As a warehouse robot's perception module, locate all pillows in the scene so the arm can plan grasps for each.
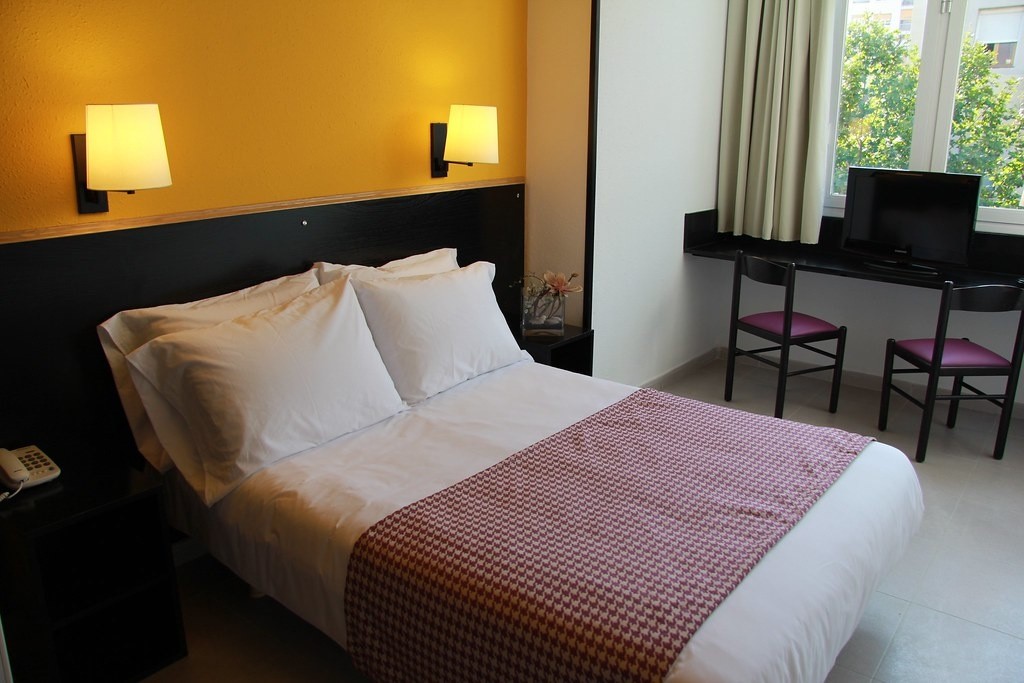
[98,267,320,477]
[123,273,409,507]
[351,263,535,404]
[313,248,460,284]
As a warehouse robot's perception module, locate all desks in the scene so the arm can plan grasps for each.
[687,207,1024,312]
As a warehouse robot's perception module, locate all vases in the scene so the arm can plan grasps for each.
[522,293,566,337]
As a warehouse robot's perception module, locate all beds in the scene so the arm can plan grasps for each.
[1,182,928,683]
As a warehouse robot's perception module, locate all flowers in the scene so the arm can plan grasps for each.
[522,268,584,327]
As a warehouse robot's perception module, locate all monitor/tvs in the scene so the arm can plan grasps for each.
[840,166,983,277]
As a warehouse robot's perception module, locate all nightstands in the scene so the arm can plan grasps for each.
[0,434,188,683]
[518,324,595,375]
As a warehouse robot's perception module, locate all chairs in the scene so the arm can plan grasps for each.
[723,250,847,422]
[878,279,1024,462]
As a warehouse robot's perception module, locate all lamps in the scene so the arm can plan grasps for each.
[430,104,501,178]
[68,102,172,213]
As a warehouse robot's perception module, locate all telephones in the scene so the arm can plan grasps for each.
[0,445,61,491]
